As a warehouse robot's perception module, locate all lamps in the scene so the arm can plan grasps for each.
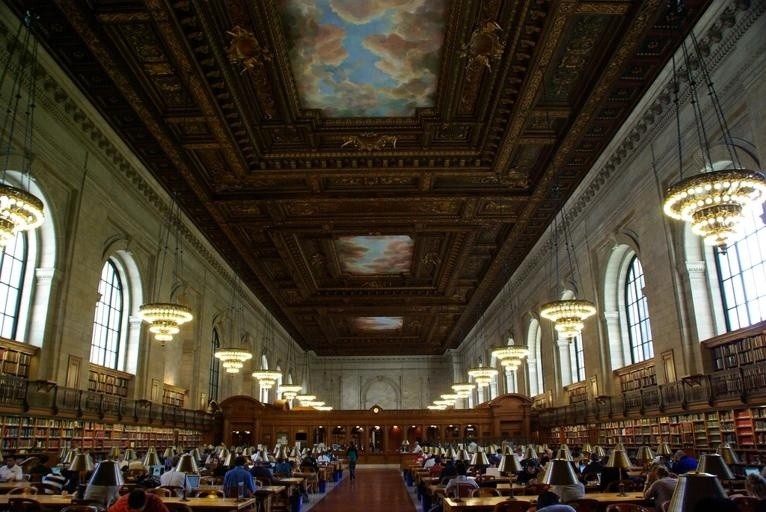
[139,192,193,347]
[0,11,46,245]
[0,442,348,512]
[540,171,597,344]
[215,348,332,416]
[400,441,766,512]
[663,29,765,255]
[427,344,530,410]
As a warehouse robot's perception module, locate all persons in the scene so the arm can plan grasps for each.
[1,439,360,511]
[402,439,766,511]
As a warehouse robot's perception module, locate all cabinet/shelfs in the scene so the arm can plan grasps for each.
[530,322,766,451]
[0,338,215,447]
